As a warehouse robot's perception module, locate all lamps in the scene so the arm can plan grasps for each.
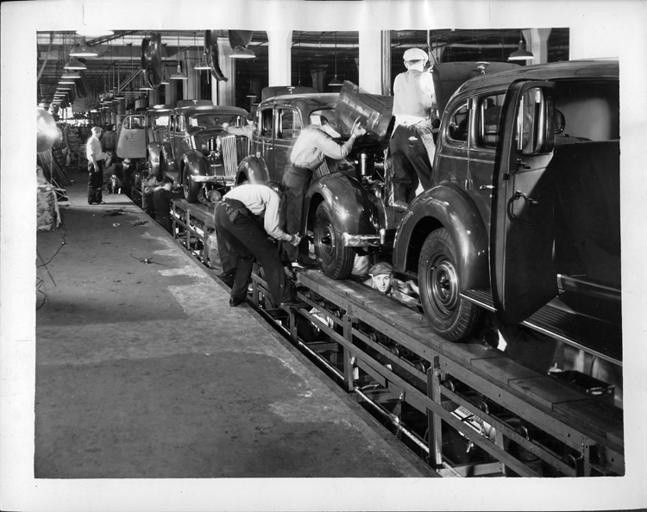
[51,14,256,97]
[328,32,344,88]
[507,30,535,62]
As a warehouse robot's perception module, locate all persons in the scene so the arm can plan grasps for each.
[386,47,440,210]
[281,109,368,269]
[85,122,132,206]
[213,181,308,308]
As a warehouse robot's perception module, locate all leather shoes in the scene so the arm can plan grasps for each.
[297,254,321,269]
[279,301,308,308]
[229,289,248,307]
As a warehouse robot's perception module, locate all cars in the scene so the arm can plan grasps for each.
[388,59,620,368]
[116,54,394,283]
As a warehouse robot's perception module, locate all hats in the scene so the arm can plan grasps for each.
[402,47,429,63]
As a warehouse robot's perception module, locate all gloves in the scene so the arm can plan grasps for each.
[290,232,301,247]
